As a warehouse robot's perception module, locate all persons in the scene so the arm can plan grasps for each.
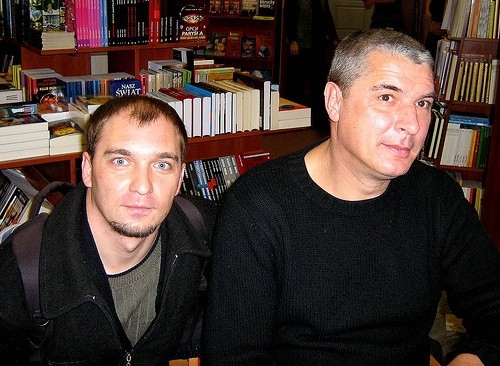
[198,26,500,366]
[0,95,216,366]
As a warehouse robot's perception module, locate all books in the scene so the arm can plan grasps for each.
[0,0,312,236]
[417,1,500,220]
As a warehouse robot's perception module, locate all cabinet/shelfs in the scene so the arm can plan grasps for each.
[0,0,500,250]
